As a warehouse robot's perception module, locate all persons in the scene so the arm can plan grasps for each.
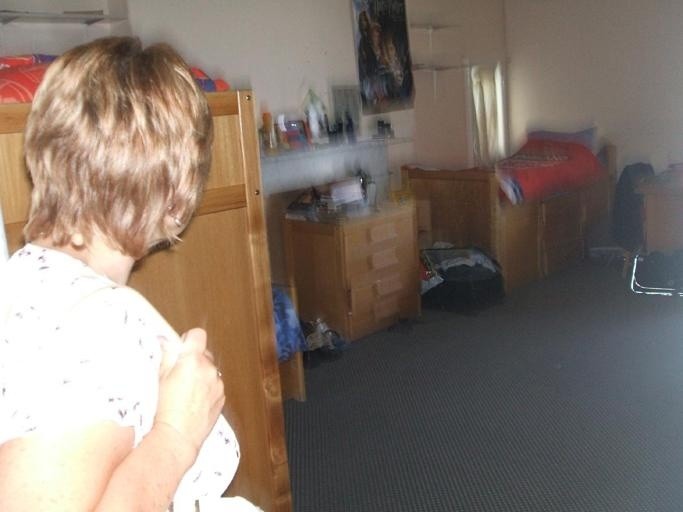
[0,34,270,512]
[356,9,373,84]
[364,22,389,84]
[380,35,406,109]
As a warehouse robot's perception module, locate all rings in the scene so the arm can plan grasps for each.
[216,366,222,376]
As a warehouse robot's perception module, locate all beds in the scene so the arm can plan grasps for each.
[401,126,615,295]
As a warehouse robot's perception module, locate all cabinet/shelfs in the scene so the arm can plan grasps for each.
[4,91,292,511]
[286,200,423,344]
[261,122,414,214]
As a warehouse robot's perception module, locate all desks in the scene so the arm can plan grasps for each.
[632,168,683,256]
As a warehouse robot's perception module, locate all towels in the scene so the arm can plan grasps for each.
[527,127,610,157]
